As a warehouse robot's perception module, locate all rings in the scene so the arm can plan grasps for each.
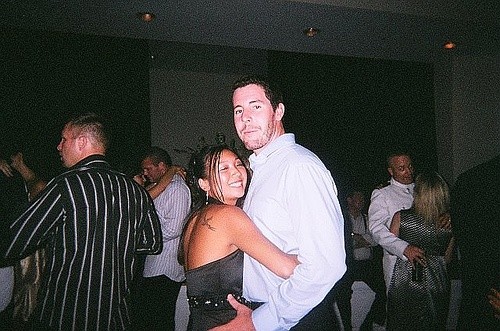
[449,219,451,222]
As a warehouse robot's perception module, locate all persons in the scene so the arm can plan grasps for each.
[0,136,62,331]
[387,169,457,331]
[336,185,388,331]
[367,148,454,289]
[0,131,24,331]
[124,159,187,200]
[126,146,191,331]
[208,75,347,331]
[0,112,163,331]
[177,142,300,331]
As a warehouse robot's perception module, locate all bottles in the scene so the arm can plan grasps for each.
[413,249,423,282]
